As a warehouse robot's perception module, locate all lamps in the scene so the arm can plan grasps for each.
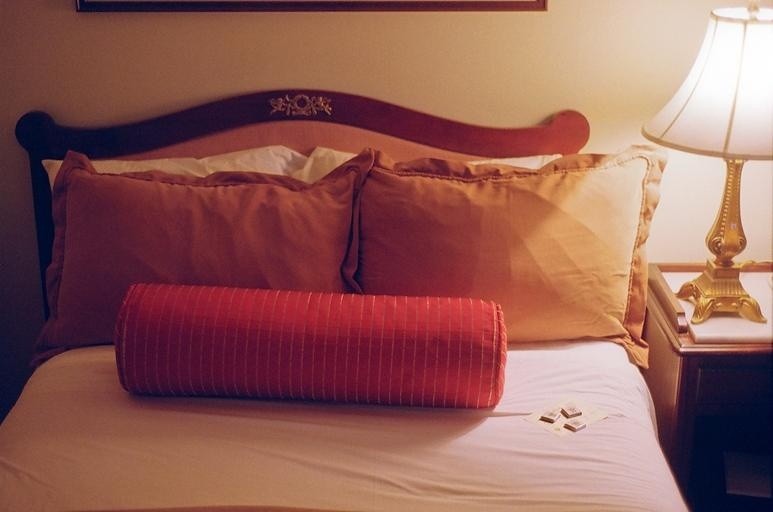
[641,7,773,324]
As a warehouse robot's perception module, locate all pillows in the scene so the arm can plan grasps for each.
[30,147,374,361]
[360,144,668,368]
[114,283,507,410]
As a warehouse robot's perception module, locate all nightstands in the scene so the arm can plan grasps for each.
[640,263,773,511]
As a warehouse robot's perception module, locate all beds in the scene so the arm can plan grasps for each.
[0,89,688,511]
